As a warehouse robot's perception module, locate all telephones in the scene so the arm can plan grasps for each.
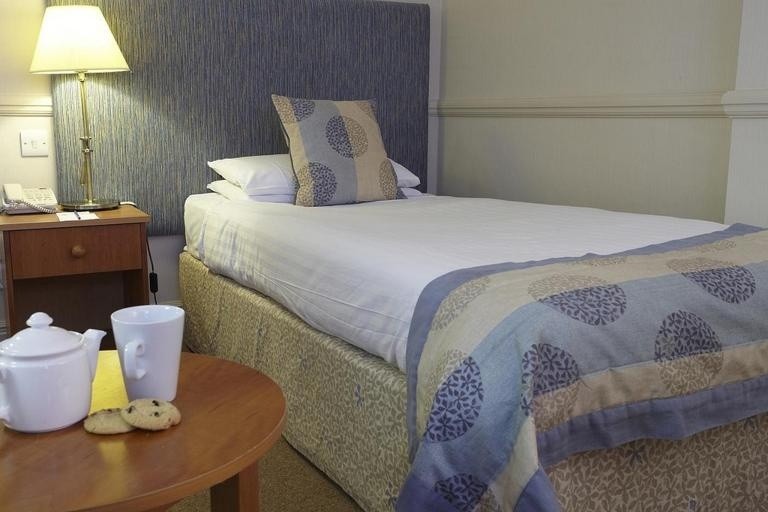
[1,182,59,215]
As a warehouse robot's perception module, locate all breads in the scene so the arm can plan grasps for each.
[122,398,181,431]
[84,408,136,435]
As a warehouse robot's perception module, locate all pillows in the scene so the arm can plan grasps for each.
[206,180,423,202]
[207,154,421,196]
[271,94,409,206]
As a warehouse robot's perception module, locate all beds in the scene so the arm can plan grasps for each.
[177,192,767,511]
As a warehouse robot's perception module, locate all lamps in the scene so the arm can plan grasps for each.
[29,4,130,211]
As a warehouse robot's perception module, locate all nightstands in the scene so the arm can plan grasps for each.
[0,205,150,338]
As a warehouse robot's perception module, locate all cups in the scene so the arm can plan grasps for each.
[110,304,185,403]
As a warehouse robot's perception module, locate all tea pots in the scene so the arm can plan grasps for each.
[0,311,107,434]
